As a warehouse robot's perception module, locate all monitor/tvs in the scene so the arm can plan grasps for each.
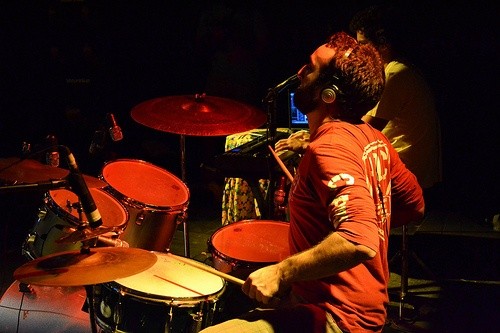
[287,87,310,129]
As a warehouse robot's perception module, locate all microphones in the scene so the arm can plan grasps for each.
[64,146,103,229]
[55,223,130,248]
[108,113,124,141]
[45,135,60,167]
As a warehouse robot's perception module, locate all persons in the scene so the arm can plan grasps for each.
[241,32,426,333]
[222,129,273,227]
[275,20,441,185]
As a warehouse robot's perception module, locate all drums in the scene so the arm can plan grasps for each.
[209,219,291,281]
[99,158,191,251]
[93,251,230,333]
[21,190,130,263]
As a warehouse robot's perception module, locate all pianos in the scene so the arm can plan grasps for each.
[216,132,306,221]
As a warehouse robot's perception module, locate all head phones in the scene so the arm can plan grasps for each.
[319,43,359,107]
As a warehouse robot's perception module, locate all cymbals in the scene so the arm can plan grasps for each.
[13,246,158,286]
[0,155,109,190]
[131,95,269,138]
